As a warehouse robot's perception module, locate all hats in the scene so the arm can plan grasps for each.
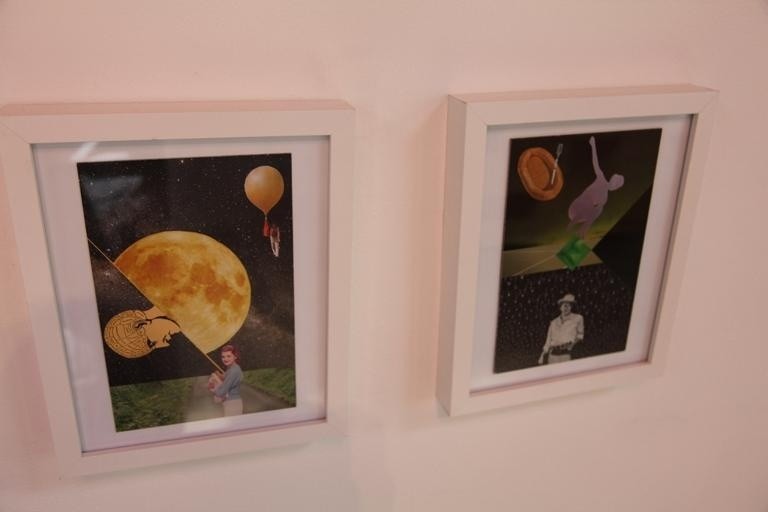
[556,294,579,306]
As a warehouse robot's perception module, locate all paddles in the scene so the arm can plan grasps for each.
[551,142,563,184]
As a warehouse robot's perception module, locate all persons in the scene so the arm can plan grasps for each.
[269,219,283,258]
[567,135,625,240]
[101,300,184,362]
[208,342,246,419]
[536,292,586,368]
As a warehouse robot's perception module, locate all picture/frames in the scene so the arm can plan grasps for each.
[0,99,357,479]
[436,85,720,418]
